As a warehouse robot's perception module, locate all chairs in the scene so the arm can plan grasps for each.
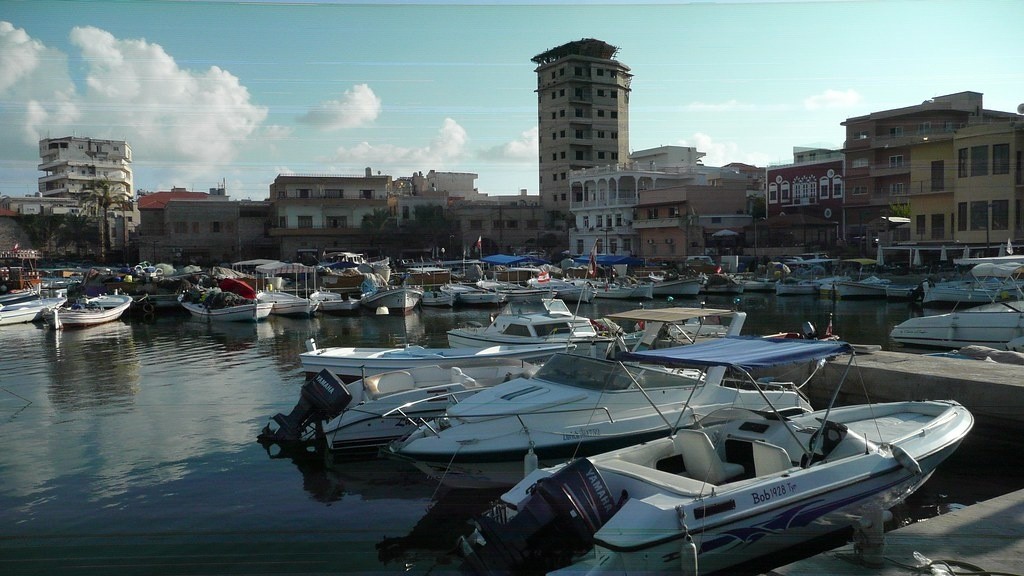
[753,439,794,478]
[677,429,744,484]
[450,367,483,389]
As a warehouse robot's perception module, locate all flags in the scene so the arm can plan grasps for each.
[476,236,482,248]
[11,243,18,252]
[538,271,550,282]
[589,244,600,275]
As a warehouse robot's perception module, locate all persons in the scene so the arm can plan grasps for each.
[101,266,148,285]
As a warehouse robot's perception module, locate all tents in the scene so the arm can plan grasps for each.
[194,253,644,298]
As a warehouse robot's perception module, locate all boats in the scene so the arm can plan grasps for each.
[400,305,815,490]
[297,336,577,389]
[603,304,736,350]
[446,294,599,361]
[254,357,548,457]
[888,262,1024,349]
[460,332,975,576]
[0,253,1024,328]
